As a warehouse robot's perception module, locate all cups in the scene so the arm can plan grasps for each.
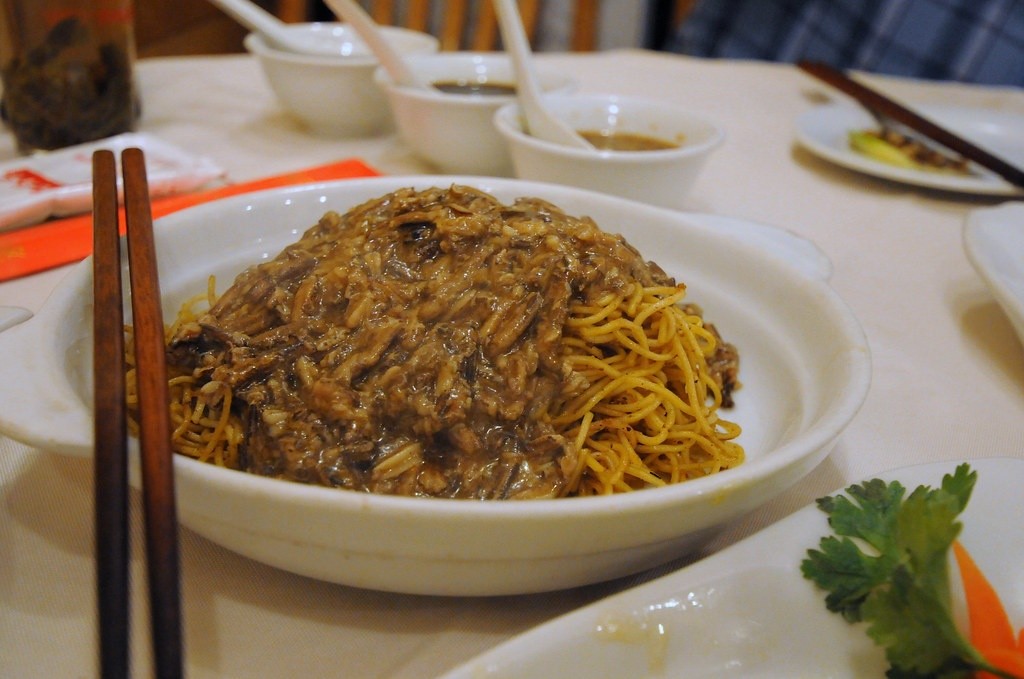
[0,0,141,154]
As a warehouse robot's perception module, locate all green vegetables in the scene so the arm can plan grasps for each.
[800,462,1024,678]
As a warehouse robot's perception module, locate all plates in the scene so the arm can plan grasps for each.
[960,201,1024,344]
[0,131,227,228]
[424,458,1024,678]
[792,102,1024,199]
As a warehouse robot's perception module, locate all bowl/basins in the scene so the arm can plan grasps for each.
[0,177,871,597]
[370,48,580,177]
[242,21,438,138]
[492,91,723,212]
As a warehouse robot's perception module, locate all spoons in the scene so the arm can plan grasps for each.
[491,0,595,151]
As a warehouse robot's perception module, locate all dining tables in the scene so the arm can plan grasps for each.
[1,53,1022,679]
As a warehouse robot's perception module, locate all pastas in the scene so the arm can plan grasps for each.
[123,269,747,496]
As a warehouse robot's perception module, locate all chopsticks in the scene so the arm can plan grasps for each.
[793,54,1024,190]
[89,147,185,679]
